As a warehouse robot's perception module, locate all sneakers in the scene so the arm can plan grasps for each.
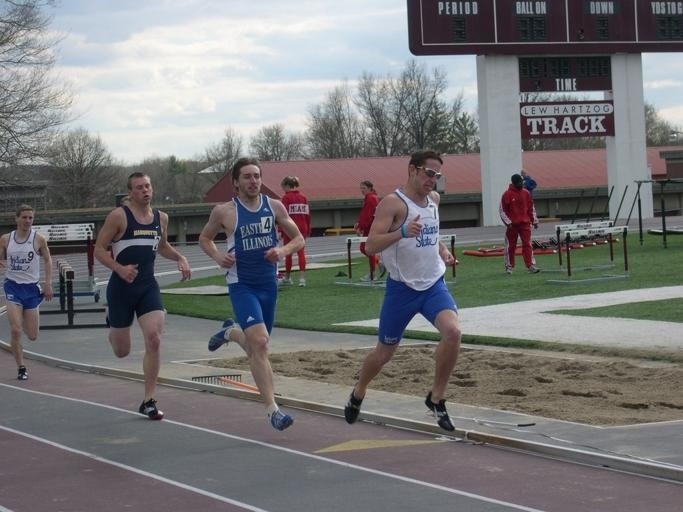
[529,267,540,274]
[505,268,511,274]
[106,307,111,327]
[18,366,29,380]
[379,263,387,279]
[425,391,455,431]
[298,279,306,287]
[277,278,293,286]
[269,407,293,432]
[360,273,377,281]
[208,318,234,351]
[139,400,163,419]
[344,382,365,425]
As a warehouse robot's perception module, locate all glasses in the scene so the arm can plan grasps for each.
[417,167,442,179]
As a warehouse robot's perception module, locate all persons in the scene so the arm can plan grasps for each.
[355,180,389,282]
[199,160,305,431]
[278,175,309,288]
[95,171,191,422]
[520,170,539,229]
[344,151,461,430]
[500,176,541,275]
[0,205,51,381]
